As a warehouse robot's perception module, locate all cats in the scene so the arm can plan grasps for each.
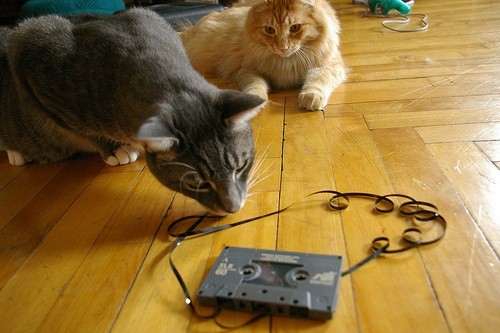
[0,7,266,215]
[174,0,347,111]
